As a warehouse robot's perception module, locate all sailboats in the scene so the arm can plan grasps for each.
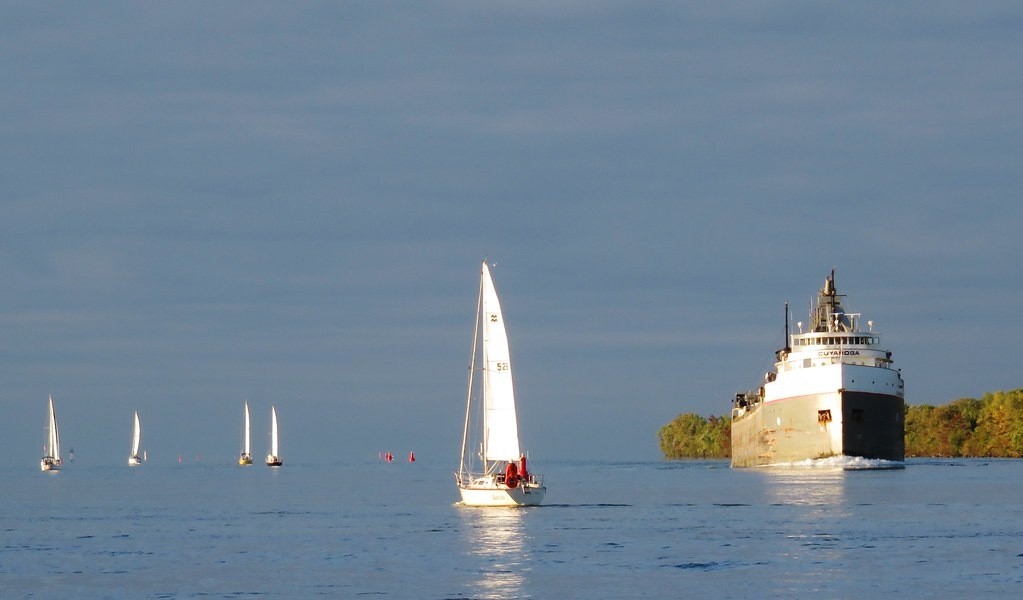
[453,257,550,510]
[263,405,285,466]
[237,399,255,466]
[39,393,62,474]
[127,410,144,465]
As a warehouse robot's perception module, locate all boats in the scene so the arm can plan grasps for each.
[729,267,907,466]
